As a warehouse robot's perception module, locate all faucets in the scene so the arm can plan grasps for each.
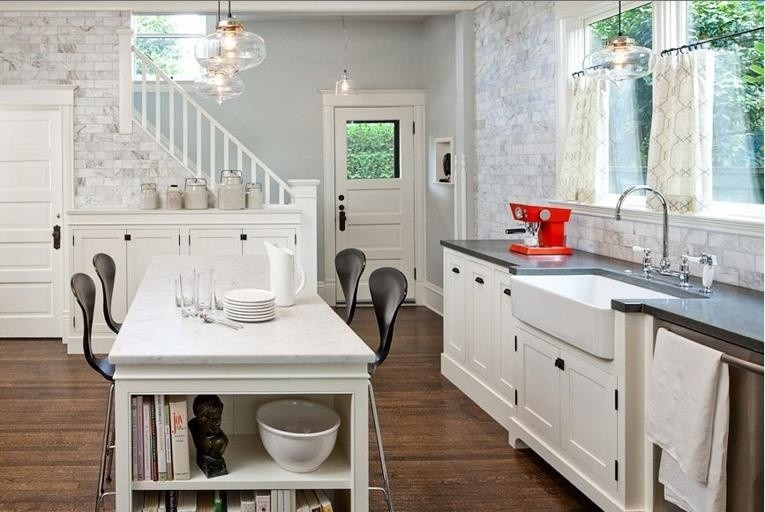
[616,184,668,259]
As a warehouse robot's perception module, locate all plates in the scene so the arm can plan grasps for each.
[222,288,277,322]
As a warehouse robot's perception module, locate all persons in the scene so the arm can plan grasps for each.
[188,395,230,461]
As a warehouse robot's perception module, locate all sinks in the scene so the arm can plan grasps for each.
[510,275,682,361]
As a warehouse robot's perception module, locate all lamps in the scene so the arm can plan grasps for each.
[334,17,358,99]
[192,1,267,109]
[581,1,657,90]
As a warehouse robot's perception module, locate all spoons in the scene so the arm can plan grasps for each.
[200,314,244,331]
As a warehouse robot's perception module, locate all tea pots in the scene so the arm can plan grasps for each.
[264,240,306,307]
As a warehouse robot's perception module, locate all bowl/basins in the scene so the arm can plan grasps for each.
[255,398,342,473]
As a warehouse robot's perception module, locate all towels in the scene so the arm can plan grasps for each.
[644,325,733,511]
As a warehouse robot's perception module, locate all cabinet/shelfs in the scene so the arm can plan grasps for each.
[512,326,623,511]
[443,249,513,449]
[66,227,298,354]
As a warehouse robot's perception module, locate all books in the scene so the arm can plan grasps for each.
[130,489,336,512]
[131,395,192,480]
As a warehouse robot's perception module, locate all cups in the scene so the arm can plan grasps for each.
[174,268,235,318]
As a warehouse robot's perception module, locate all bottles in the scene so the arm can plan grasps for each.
[166,185,183,210]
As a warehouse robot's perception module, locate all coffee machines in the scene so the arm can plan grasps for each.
[505,203,573,256]
